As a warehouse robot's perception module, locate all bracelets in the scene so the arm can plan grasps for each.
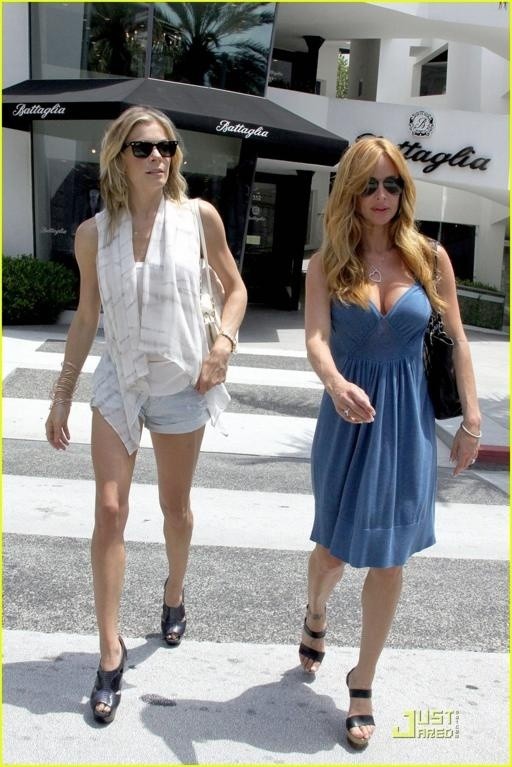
[48,360,81,409]
[460,422,482,439]
[219,331,237,352]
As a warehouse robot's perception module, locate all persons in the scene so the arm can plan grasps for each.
[44,106,248,725]
[299,137,481,751]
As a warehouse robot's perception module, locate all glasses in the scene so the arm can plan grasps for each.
[359,175,404,198]
[123,140,178,158]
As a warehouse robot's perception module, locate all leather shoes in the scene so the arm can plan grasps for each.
[91,638,128,723]
[299,605,328,674]
[346,669,374,751]
[161,576,186,645]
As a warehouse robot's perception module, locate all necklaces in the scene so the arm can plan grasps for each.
[132,228,146,239]
[363,252,386,283]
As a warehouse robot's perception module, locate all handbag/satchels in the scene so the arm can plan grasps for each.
[422,310,463,419]
[201,264,239,354]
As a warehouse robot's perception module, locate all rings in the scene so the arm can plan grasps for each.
[471,458,475,465]
[343,408,350,417]
